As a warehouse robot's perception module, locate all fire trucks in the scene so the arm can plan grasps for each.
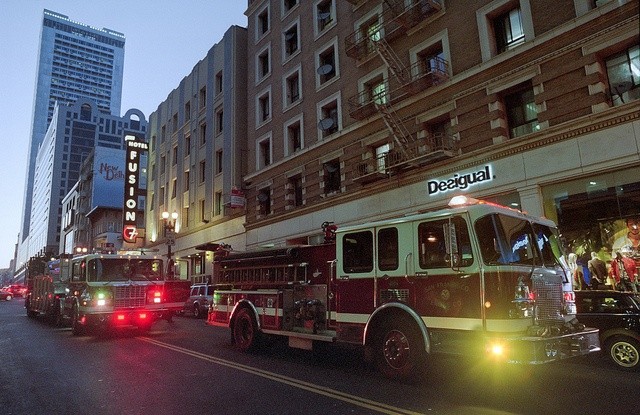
[202,194,601,383]
[22,254,187,342]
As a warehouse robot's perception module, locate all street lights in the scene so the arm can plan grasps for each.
[162,212,178,280]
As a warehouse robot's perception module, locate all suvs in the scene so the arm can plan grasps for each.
[175,282,214,319]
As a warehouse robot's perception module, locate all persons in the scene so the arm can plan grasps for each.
[589,251,608,289]
[568,253,577,293]
[610,250,639,291]
